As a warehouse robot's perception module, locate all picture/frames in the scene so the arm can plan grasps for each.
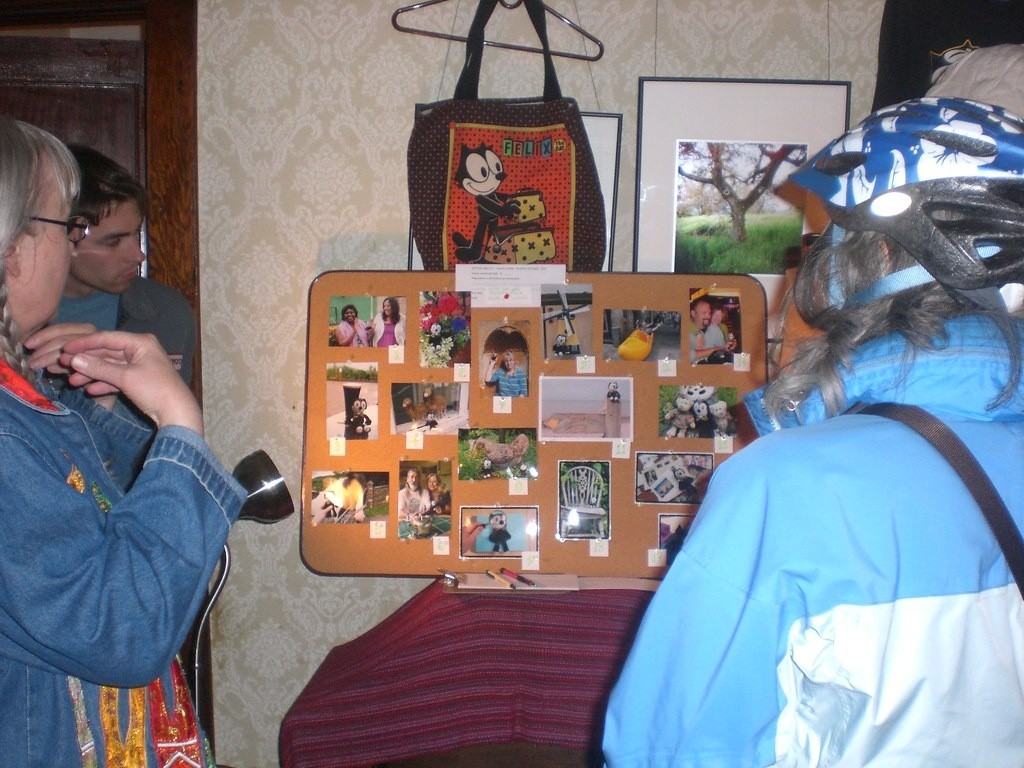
[406,103,624,274]
[632,75,853,271]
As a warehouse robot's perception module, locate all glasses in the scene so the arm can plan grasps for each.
[30,214,89,242]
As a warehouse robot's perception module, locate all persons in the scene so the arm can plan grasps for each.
[369,297,407,347]
[462,522,483,554]
[426,473,452,514]
[48,143,195,387]
[312,498,333,523]
[335,304,376,347]
[689,299,737,365]
[398,467,432,520]
[485,351,528,397]
[601,97,1024,768]
[0,115,246,767]
[635,318,662,335]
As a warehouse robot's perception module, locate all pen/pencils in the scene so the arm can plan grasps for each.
[499,568,536,586]
[485,570,516,589]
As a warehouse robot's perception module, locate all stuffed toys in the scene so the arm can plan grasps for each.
[661,384,738,439]
[553,334,568,357]
[345,399,372,433]
[607,382,621,403]
[428,323,443,350]
[480,458,495,479]
[674,468,698,495]
[426,412,438,429]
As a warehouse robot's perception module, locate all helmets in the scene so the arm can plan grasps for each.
[789,96,1024,289]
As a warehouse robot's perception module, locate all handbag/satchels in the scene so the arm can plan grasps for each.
[407,1,606,271]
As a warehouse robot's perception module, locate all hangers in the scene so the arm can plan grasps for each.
[391,0,604,60]
